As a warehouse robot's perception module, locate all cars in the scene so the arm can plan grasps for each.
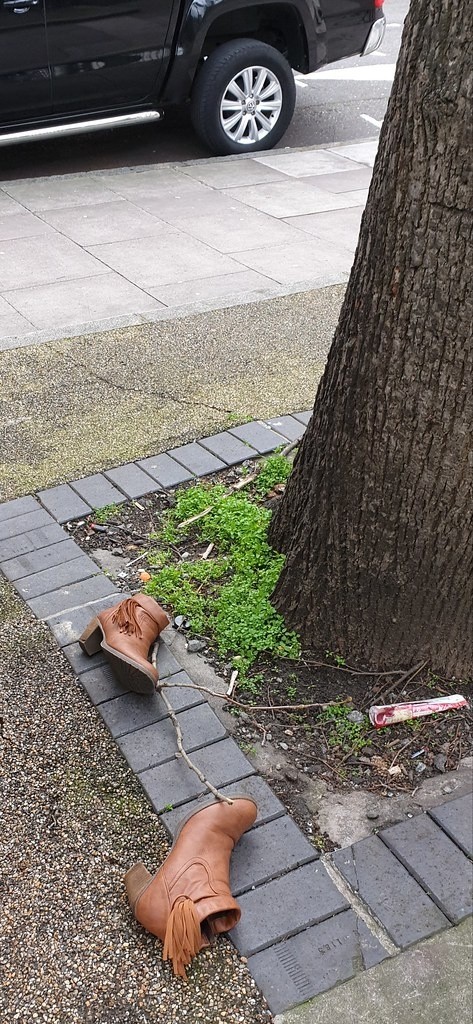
[0,0,386,158]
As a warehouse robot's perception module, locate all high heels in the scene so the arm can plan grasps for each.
[78,592,170,695]
[125,793,258,979]
